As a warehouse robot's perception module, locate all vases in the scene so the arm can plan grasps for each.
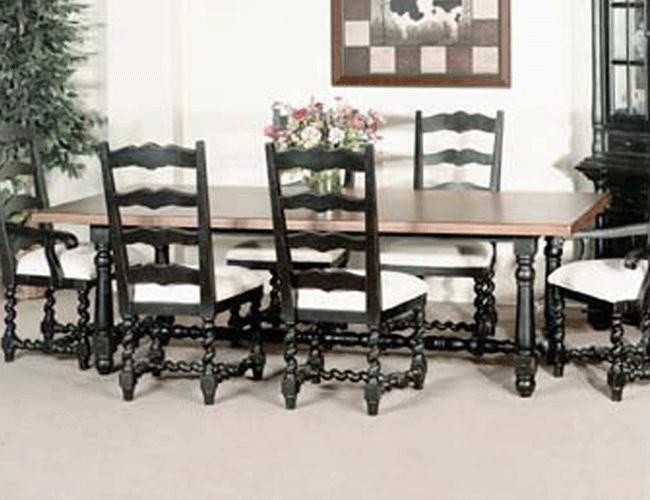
[302,166,344,215]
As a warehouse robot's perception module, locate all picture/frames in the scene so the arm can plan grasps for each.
[330,0,516,89]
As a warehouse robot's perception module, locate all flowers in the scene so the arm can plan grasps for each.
[262,95,384,151]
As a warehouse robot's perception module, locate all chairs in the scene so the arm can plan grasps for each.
[223,168,352,348]
[96,138,271,404]
[265,142,428,414]
[1,121,140,369]
[363,110,505,357]
[546,256,648,402]
